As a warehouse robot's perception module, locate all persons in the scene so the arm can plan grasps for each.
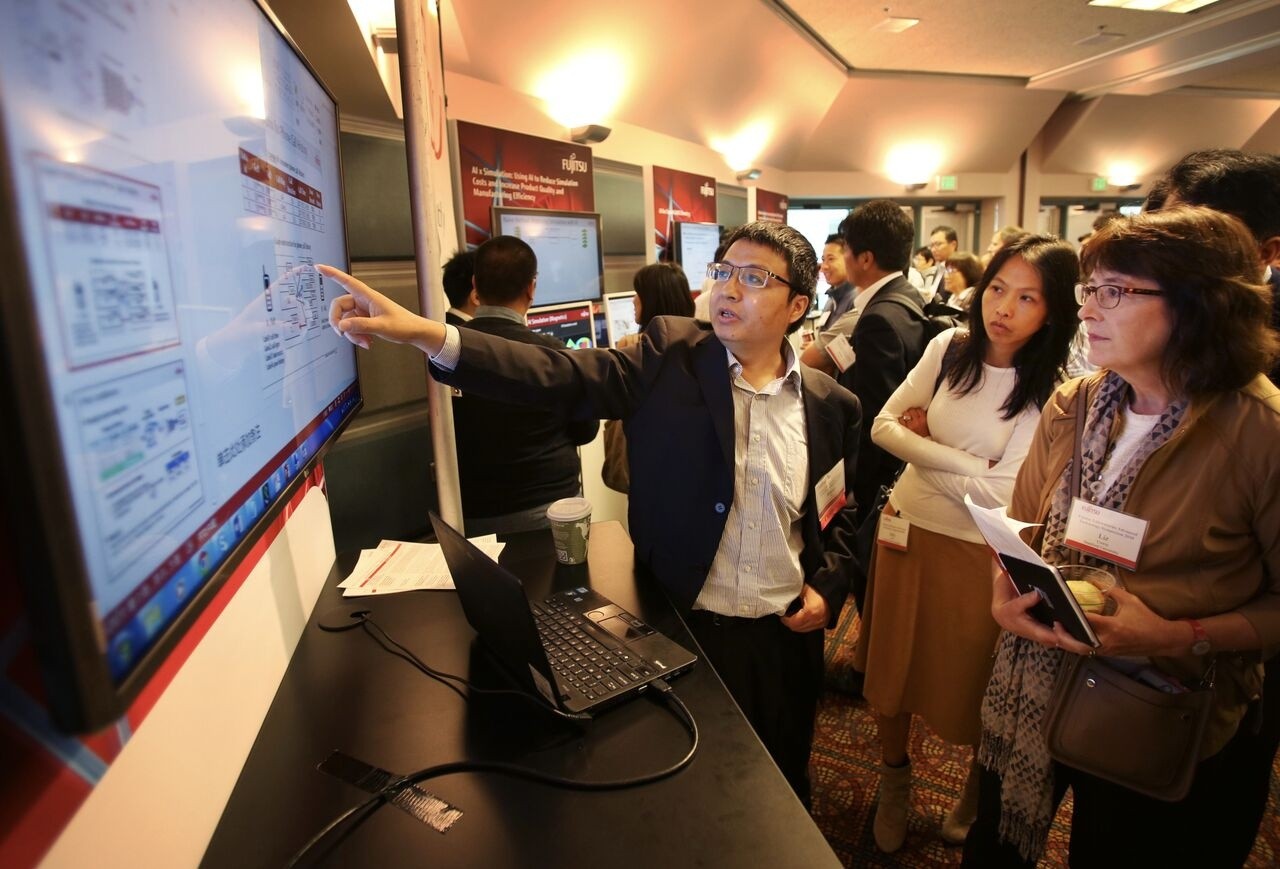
[315,201,928,815]
[906,147,1280,392]
[959,206,1280,869]
[853,233,1083,855]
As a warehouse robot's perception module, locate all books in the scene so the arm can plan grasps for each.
[997,552,1102,650]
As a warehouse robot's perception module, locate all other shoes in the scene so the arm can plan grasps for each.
[833,660,865,701]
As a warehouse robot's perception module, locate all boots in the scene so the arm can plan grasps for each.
[941,760,987,846]
[875,758,912,852]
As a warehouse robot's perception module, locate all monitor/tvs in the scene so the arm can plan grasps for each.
[671,221,725,290]
[1,0,364,732]
[489,205,640,350]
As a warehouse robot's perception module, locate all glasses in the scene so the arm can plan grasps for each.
[1075,282,1167,309]
[942,268,960,275]
[706,260,796,290]
[928,242,953,249]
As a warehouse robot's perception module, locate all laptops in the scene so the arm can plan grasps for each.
[427,512,696,721]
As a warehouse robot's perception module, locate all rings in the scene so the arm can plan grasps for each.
[903,415,911,422]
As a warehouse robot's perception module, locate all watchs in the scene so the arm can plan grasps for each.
[1186,619,1213,658]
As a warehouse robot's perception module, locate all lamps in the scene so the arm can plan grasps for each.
[736,169,761,181]
[571,124,610,143]
[907,183,926,192]
[373,27,399,55]
[1119,185,1142,194]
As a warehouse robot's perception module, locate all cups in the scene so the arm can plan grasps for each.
[546,497,592,565]
[1054,565,1116,632]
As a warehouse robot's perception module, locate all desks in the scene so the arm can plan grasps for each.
[197,519,846,869]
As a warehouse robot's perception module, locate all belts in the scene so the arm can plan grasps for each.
[685,610,781,626]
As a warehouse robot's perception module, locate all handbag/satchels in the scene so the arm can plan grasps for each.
[601,334,640,496]
[1046,654,1211,802]
